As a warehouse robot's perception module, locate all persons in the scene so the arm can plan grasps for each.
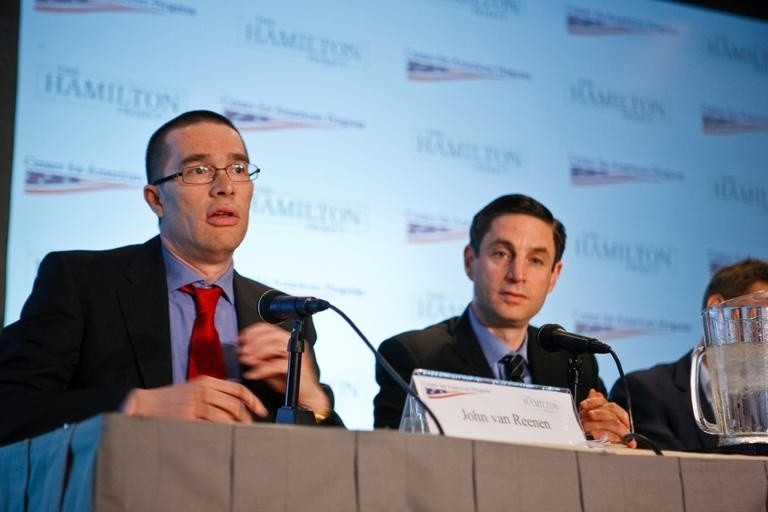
[609,259,768,453]
[0,110,346,446]
[373,194,637,448]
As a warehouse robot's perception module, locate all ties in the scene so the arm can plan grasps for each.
[498,352,529,384]
[178,280,230,381]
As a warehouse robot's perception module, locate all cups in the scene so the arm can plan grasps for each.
[690,291,768,449]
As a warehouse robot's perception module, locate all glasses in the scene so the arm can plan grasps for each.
[152,160,261,186]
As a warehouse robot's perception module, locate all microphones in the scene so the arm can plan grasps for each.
[255,288,329,326]
[535,323,609,355]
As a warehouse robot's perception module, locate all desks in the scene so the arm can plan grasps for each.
[2,411,768,512]
